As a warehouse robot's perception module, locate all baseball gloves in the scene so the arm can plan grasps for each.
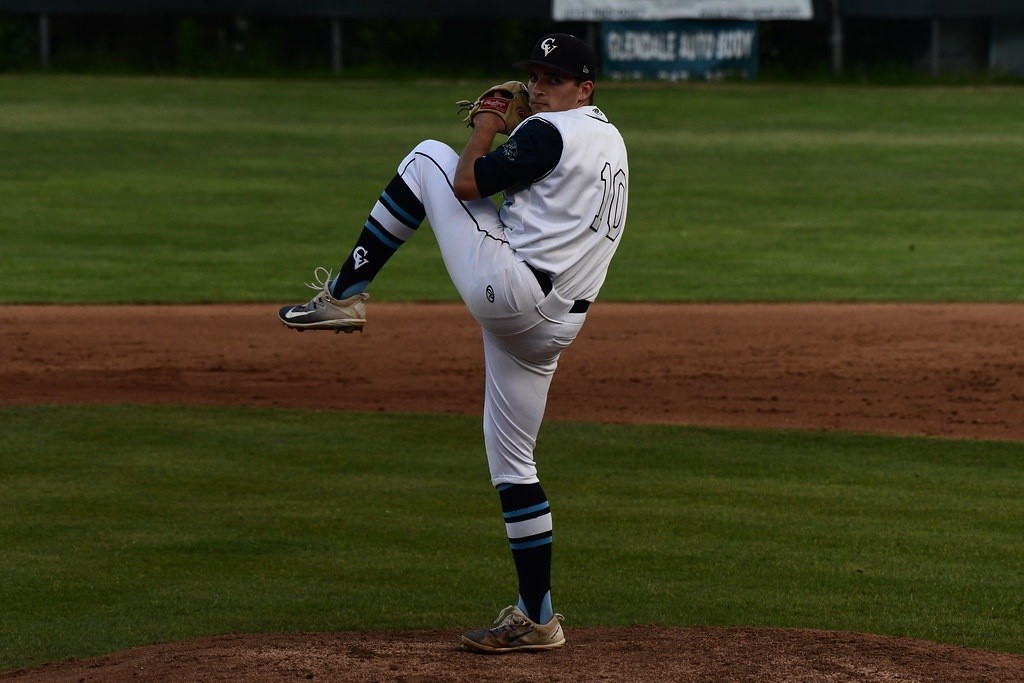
[466,80,529,135]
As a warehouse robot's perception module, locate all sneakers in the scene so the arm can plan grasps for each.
[462,604,566,651]
[277,266,370,334]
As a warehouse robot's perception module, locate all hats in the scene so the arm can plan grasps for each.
[517,33,596,81]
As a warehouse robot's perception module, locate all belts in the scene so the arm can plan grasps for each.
[524,260,591,313]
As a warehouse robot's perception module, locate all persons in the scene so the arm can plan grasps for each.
[277,32,630,655]
